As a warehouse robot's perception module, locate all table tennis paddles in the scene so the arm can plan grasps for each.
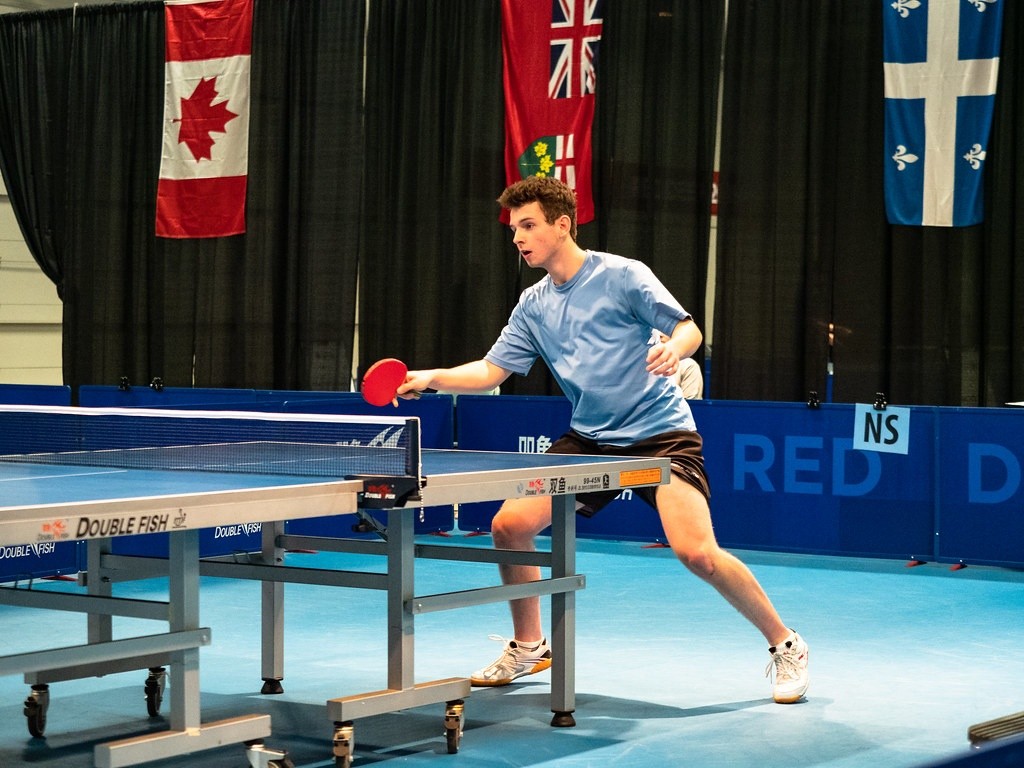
[362,357,425,406]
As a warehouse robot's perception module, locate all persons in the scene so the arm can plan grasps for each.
[655,328,704,401]
[389,176,812,704]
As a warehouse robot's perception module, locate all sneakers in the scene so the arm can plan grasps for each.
[765,629,809,702]
[471,633,553,685]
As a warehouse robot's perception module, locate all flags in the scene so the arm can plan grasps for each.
[499,1,603,227]
[881,0,1003,227]
[154,1,254,238]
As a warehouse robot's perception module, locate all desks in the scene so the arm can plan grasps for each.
[1,404,671,768]
